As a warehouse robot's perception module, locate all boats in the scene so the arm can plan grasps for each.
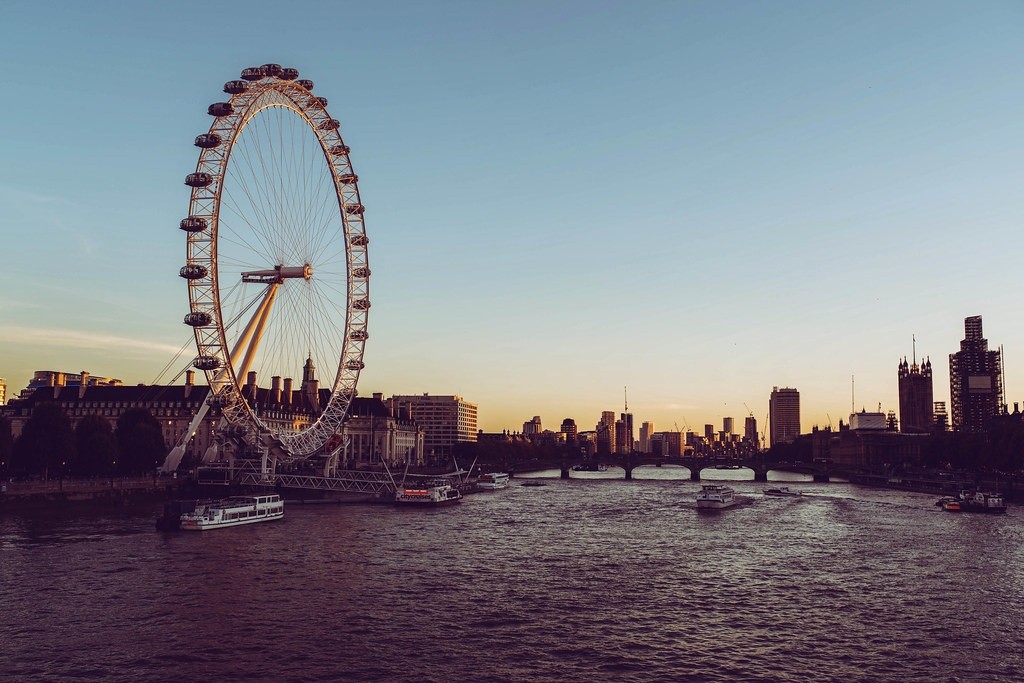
[477,472,511,490]
[696,484,736,513]
[178,492,286,531]
[394,484,464,508]
[935,484,1008,513]
[762,486,803,496]
[572,462,607,472]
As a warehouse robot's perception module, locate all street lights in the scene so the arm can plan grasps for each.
[0,461,7,493]
[59,461,66,493]
[153,460,158,488]
[111,460,116,489]
